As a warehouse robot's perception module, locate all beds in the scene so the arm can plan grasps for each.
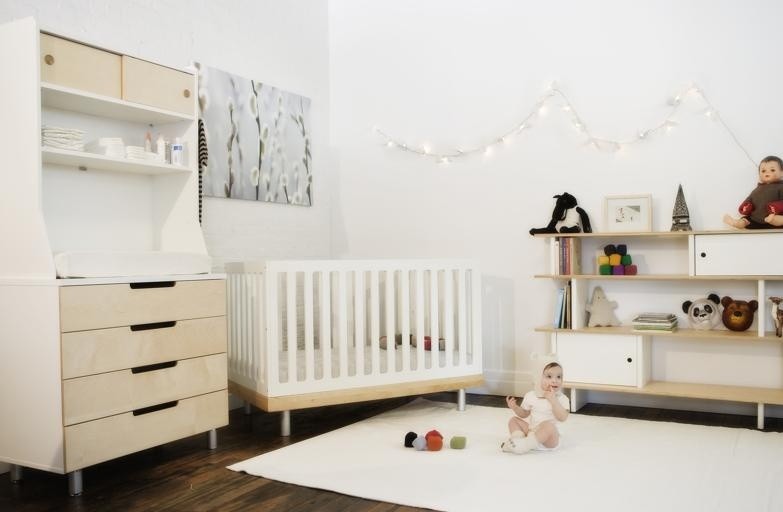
[226,255,483,438]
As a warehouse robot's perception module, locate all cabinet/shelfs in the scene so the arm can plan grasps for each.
[0,15,228,497]
[531,227,783,430]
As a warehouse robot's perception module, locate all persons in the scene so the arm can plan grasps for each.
[499,350,569,452]
[723,156,783,229]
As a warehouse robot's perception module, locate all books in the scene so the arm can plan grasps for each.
[553,280,572,329]
[550,236,581,274]
[632,313,678,333]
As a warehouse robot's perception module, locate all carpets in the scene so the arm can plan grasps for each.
[225,397,783,511]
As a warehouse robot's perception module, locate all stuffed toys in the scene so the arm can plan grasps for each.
[584,286,621,328]
[682,293,722,332]
[720,296,758,332]
[529,192,592,234]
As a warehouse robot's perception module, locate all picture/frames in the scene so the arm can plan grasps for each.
[605,193,652,232]
